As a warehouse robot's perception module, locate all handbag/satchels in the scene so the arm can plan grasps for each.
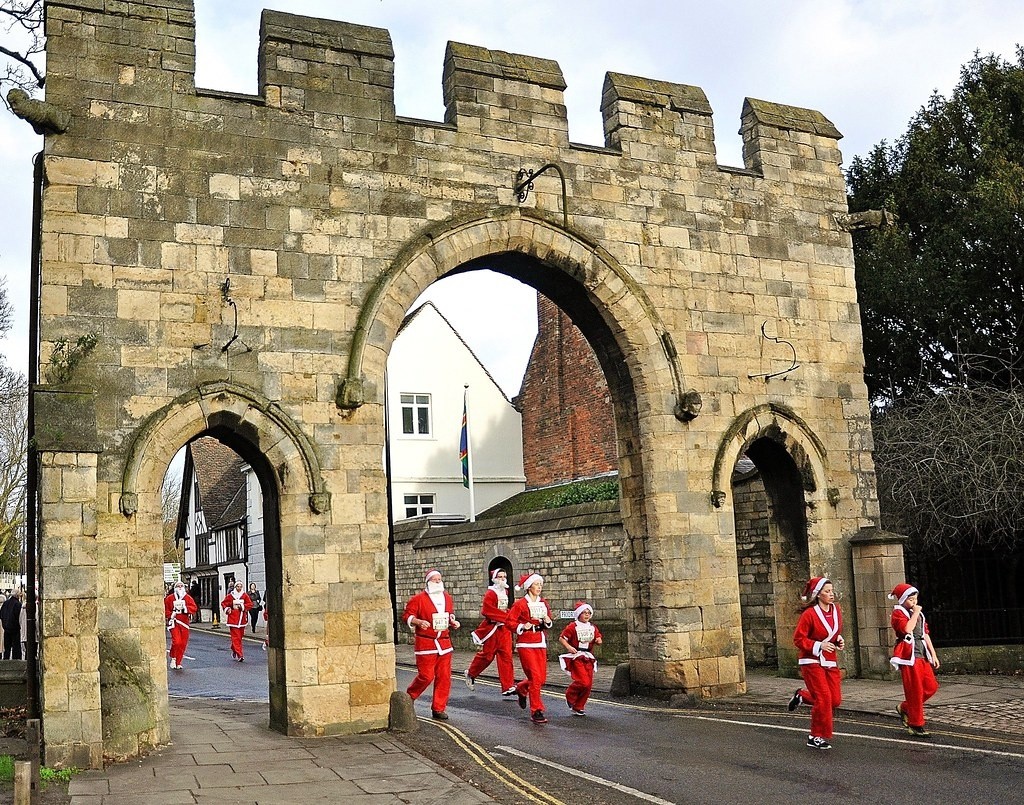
[258,605,264,611]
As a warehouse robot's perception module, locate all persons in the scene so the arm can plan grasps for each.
[227,582,234,595]
[402,569,460,719]
[230,575,236,584]
[248,583,261,633]
[463,568,517,696]
[19,591,38,659]
[164,582,197,669]
[221,580,253,662]
[169,584,174,594]
[190,580,201,622]
[787,577,845,749]
[504,573,553,723]
[262,589,269,650]
[888,584,940,738]
[559,602,602,715]
[0,589,22,659]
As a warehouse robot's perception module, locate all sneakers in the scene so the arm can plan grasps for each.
[502,687,518,695]
[806,734,832,749]
[907,726,932,738]
[530,709,548,723]
[465,669,474,690]
[787,687,804,712]
[569,706,586,716]
[565,696,572,709]
[516,688,527,710]
[895,704,909,727]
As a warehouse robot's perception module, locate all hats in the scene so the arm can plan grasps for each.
[573,602,594,618]
[173,581,185,590]
[424,568,441,583]
[514,572,544,591]
[489,567,504,579]
[801,576,830,603]
[887,583,919,604]
[233,580,243,587]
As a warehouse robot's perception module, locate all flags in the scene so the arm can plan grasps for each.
[459,390,469,489]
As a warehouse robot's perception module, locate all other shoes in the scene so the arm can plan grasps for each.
[431,709,449,720]
[238,656,245,663]
[232,647,236,658]
[169,657,176,669]
[176,664,183,669]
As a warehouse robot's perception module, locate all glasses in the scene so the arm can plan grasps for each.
[582,610,592,615]
